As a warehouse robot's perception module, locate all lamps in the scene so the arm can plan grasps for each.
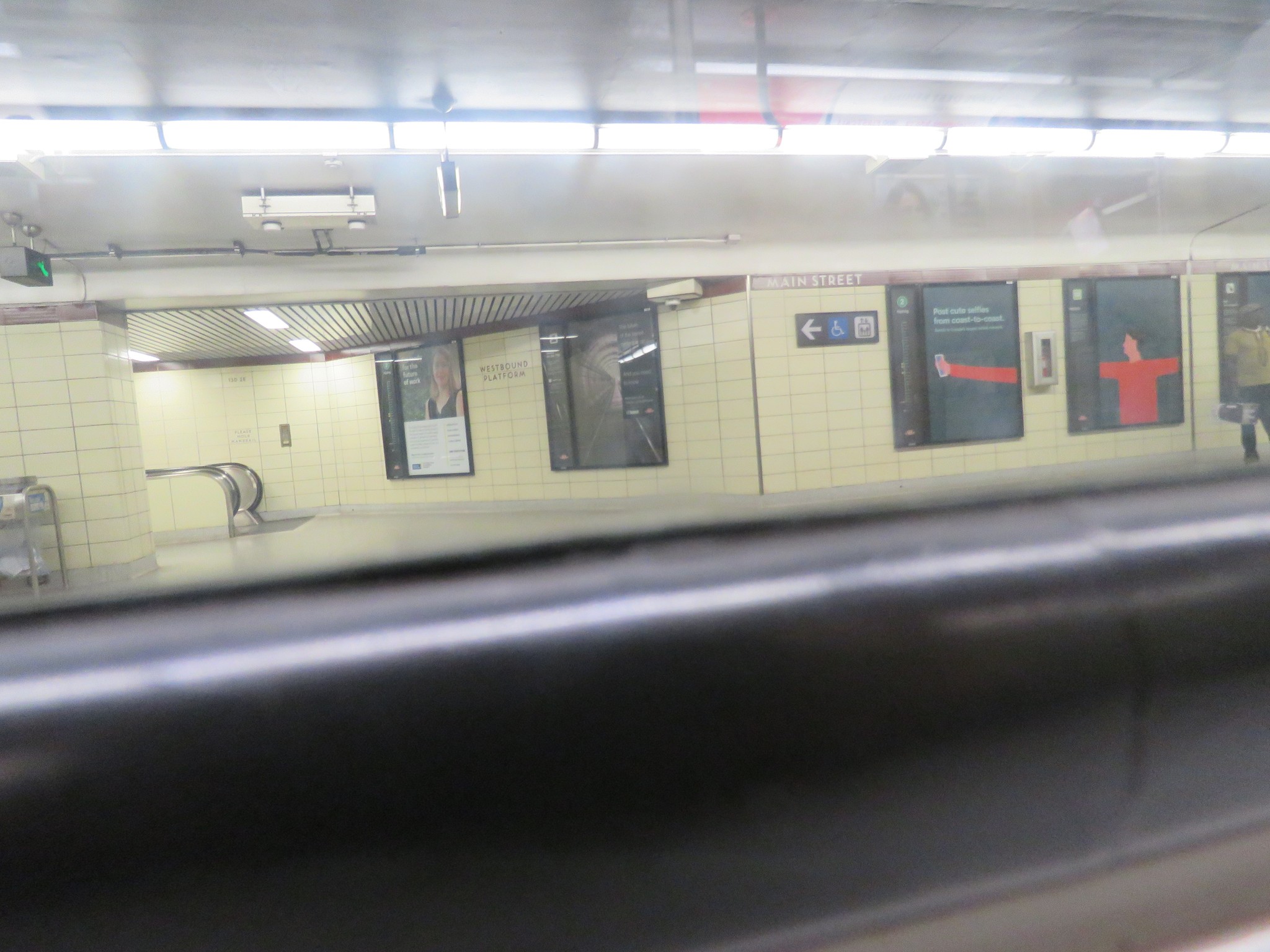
[0,101,1270,166]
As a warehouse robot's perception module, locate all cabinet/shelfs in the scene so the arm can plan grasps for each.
[0,473,69,598]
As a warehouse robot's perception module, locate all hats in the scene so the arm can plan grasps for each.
[1238,303,1263,314]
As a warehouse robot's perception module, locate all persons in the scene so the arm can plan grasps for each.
[1222,303,1270,461]
[424,348,464,420]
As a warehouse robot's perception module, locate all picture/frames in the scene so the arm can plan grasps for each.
[886,279,1024,449]
[1216,271,1269,405]
[1063,273,1185,432]
[539,301,669,471]
[374,336,475,480]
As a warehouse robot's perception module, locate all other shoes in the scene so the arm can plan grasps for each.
[1243,448,1260,464]
